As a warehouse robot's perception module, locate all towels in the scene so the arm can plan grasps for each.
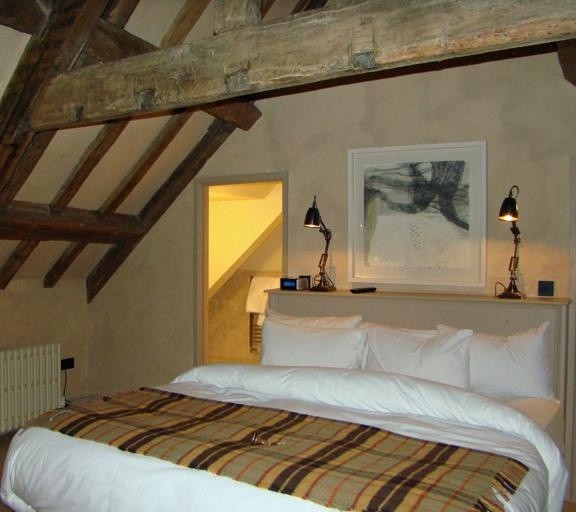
[246,277,280,327]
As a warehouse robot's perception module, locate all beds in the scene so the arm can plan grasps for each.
[1,288,576,512]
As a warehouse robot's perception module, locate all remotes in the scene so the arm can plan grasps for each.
[350,287,377,294]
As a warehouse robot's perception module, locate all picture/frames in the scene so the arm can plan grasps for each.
[348,141,487,288]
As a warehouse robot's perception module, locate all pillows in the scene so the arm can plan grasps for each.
[260,310,560,401]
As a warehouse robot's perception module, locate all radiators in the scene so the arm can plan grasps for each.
[0,343,60,434]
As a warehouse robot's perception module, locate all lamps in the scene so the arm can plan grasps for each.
[498,185,525,299]
[303,195,337,292]
